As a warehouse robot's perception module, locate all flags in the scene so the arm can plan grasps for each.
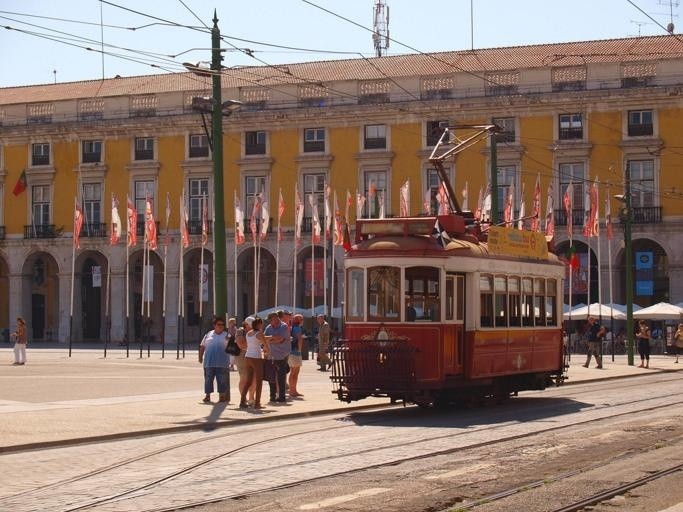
[543,182,556,252]
[182,190,191,250]
[321,183,331,239]
[342,189,355,252]
[517,183,527,229]
[436,181,452,215]
[12,169,28,199]
[474,185,483,220]
[248,195,260,242]
[603,191,613,240]
[482,182,492,223]
[274,189,289,241]
[147,222,158,252]
[461,181,470,212]
[363,181,375,213]
[332,191,345,247]
[201,191,209,245]
[142,197,155,244]
[530,173,542,236]
[258,194,271,241]
[292,185,307,248]
[308,189,322,242]
[562,181,576,239]
[178,196,189,249]
[503,181,515,227]
[73,201,85,249]
[373,191,386,217]
[110,195,121,246]
[160,192,173,248]
[126,196,137,250]
[422,188,433,216]
[354,193,365,242]
[233,191,246,245]
[568,251,580,271]
[582,173,602,237]
[397,180,411,218]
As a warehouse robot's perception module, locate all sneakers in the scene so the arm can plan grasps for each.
[316,363,332,371]
[582,365,602,368]
[674,362,678,363]
[240,383,305,409]
[638,365,649,368]
[13,362,24,365]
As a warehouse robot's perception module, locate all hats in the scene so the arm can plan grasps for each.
[265,309,293,321]
[245,317,256,329]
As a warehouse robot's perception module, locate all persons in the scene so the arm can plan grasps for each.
[9,317,27,365]
[581,315,605,370]
[672,324,682,363]
[633,319,651,369]
[316,315,333,371]
[198,317,229,404]
[226,308,305,411]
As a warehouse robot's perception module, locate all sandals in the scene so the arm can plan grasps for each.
[203,396,225,402]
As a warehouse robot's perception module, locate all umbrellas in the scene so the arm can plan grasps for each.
[246,298,429,331]
[499,293,682,326]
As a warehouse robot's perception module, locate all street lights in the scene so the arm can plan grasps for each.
[613,158,634,366]
[182,9,243,333]
[481,124,512,226]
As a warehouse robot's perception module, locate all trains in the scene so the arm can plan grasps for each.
[328,120,569,411]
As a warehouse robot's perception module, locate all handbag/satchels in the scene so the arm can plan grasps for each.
[225,337,241,357]
